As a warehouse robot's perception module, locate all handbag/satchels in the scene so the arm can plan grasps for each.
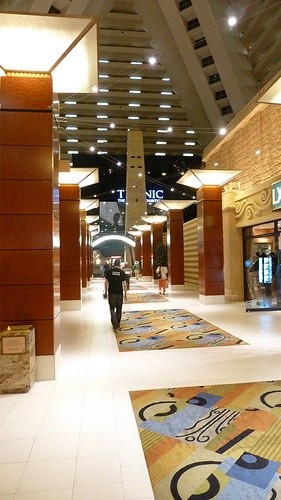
[154,265,161,279]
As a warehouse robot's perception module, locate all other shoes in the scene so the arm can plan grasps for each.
[163,293,165,295]
[112,318,120,329]
[159,292,161,294]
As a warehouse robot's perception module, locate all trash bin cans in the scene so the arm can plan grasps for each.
[0,324,35,395]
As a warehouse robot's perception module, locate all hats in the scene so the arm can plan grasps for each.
[134,261,139,265]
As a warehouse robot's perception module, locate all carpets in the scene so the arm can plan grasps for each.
[129,380,281,500]
[113,309,251,352]
[122,280,149,291]
[122,292,171,304]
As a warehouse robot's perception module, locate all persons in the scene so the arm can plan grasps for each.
[102,259,128,329]
[155,260,168,295]
[100,262,110,278]
[133,261,139,279]
